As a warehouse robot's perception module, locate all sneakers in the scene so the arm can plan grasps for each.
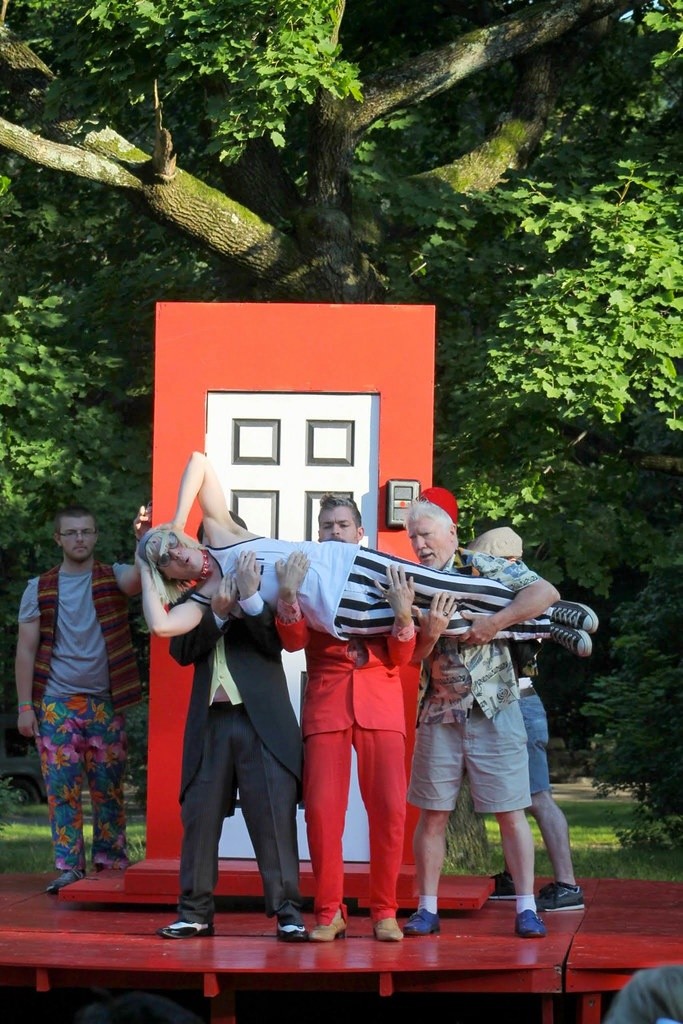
[552,621,593,657]
[487,872,517,900]
[46,868,86,894]
[535,881,585,911]
[550,598,598,633]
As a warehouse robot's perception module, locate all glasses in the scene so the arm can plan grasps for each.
[59,528,98,538]
[157,531,179,569]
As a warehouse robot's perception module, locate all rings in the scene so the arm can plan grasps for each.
[389,583,393,586]
[443,611,448,616]
[383,590,388,594]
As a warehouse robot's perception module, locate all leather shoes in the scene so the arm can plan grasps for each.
[277,921,307,942]
[404,910,440,935]
[156,917,215,938]
[515,910,545,937]
[374,919,403,941]
[308,918,346,941]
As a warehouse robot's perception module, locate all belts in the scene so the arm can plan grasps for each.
[519,686,537,697]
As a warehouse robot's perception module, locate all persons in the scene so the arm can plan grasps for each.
[466,527,585,912]
[274,491,418,941]
[401,484,560,937]
[15,505,152,893]
[73,986,204,1024]
[136,451,599,658]
[602,966,683,1024]
[156,510,311,942]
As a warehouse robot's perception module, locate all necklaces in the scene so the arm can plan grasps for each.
[192,550,209,582]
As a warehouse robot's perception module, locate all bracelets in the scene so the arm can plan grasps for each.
[17,701,33,713]
[138,539,140,542]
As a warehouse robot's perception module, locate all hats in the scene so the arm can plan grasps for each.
[420,486,459,523]
